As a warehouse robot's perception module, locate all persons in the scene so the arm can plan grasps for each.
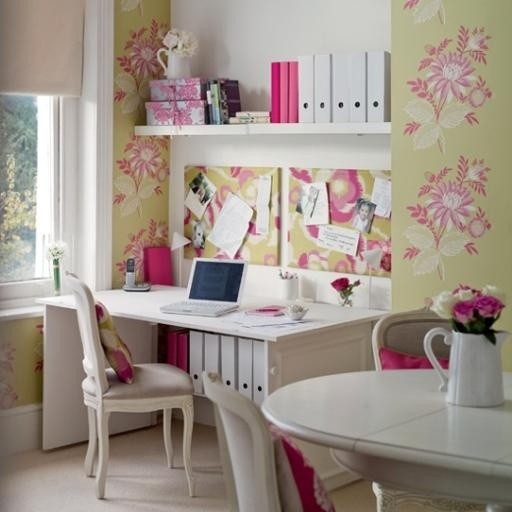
[354,201,370,232]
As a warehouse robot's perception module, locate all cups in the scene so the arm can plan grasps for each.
[281,279,298,301]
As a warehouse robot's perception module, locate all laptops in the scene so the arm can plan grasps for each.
[159,257,248,318]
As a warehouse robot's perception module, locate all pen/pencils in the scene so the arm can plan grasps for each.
[255,309,278,312]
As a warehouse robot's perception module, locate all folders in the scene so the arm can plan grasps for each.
[298,51,390,123]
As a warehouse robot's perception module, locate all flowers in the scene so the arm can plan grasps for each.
[330,277,361,306]
[46,240,65,257]
[160,26,198,57]
[431,284,505,333]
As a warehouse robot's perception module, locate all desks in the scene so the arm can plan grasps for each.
[42,283,388,495]
[261,369,512,512]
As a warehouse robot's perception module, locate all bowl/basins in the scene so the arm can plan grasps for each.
[284,309,308,320]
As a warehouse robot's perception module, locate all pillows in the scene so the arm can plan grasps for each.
[378,347,447,369]
[272,425,333,512]
[95,301,133,387]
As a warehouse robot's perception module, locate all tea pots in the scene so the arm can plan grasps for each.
[157,47,194,80]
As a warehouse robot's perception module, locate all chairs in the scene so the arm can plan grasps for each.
[201,372,335,512]
[372,309,495,512]
[62,269,196,500]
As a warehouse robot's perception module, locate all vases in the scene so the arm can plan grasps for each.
[156,50,191,77]
[53,258,60,292]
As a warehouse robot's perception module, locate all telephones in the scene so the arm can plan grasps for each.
[124,257,150,291]
